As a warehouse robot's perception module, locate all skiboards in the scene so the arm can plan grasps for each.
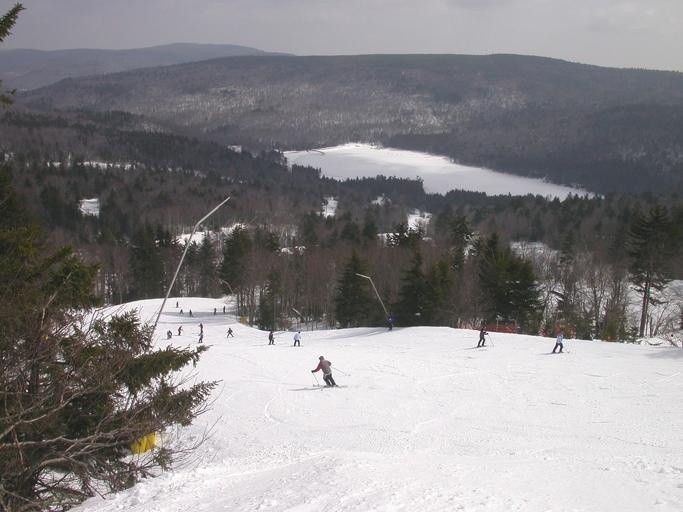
[540,351,564,355]
[464,345,488,349]
[313,383,346,389]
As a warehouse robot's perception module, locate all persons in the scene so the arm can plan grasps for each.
[311,355,335,386]
[552,331,564,353]
[166,302,234,343]
[268,331,274,344]
[387,313,393,331]
[294,331,301,346]
[477,325,489,347]
